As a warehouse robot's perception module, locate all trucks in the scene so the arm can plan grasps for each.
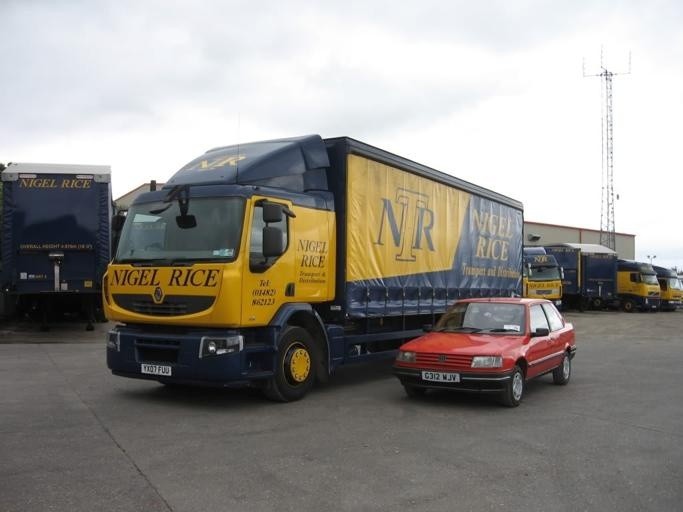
[0,162,114,323]
[101,134,524,404]
[522,242,683,312]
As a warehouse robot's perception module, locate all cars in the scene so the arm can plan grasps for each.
[390,297,577,408]
